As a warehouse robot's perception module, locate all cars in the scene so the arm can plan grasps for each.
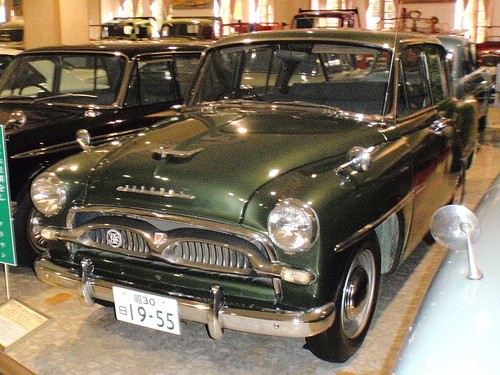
[24,31,500,364]
[0,40,278,270]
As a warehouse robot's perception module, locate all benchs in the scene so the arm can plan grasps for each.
[291,81,405,115]
